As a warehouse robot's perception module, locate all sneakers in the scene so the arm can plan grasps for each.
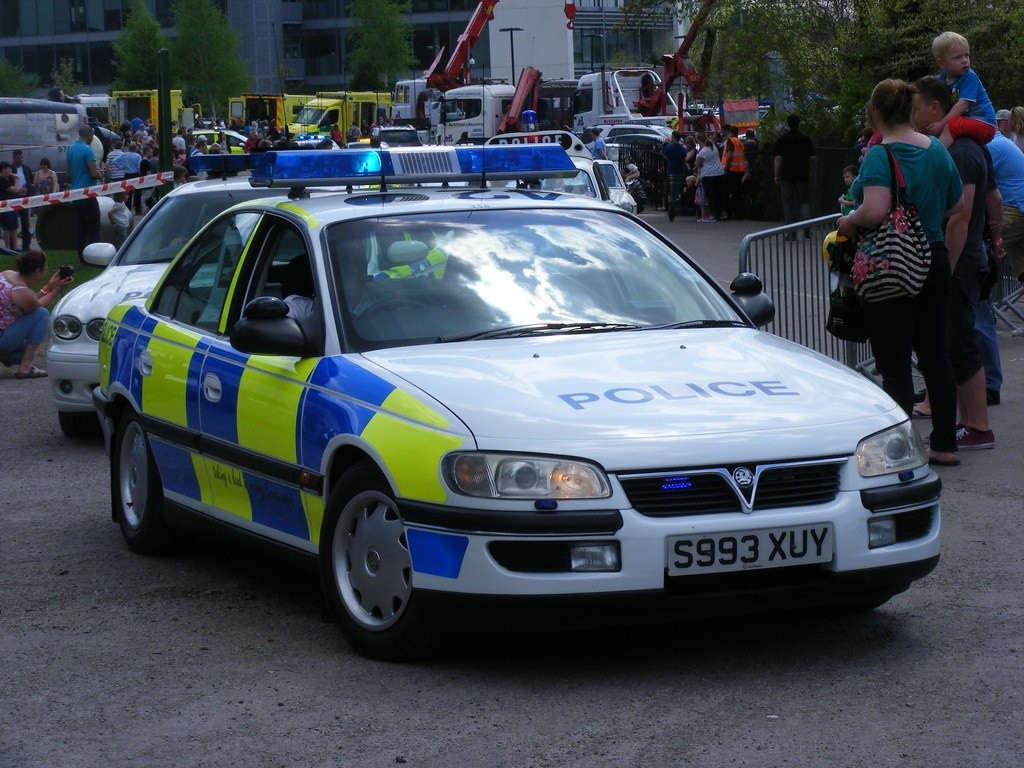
[956,423,995,450]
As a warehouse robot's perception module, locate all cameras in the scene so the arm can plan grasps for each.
[60,265,75,278]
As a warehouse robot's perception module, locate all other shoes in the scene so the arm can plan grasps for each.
[719,215,728,220]
[701,218,716,223]
[696,219,702,222]
[914,388,926,403]
[986,387,1000,405]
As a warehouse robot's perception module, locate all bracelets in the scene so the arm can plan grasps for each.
[39,285,49,295]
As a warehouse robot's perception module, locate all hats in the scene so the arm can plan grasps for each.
[823,230,848,267]
[198,135,207,141]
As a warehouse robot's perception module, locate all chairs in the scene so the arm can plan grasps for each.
[275,236,605,307]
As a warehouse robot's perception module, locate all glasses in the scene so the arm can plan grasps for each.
[685,142,691,144]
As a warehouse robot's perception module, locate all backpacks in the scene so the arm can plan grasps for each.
[695,185,708,207]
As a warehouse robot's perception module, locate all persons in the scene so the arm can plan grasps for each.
[48,86,64,103]
[283,252,493,333]
[92,112,391,245]
[563,113,816,240]
[65,123,103,261]
[0,251,74,379]
[0,150,57,252]
[835,32,1024,466]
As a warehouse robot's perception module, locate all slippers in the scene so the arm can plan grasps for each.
[929,456,960,466]
[912,409,932,419]
[14,366,48,379]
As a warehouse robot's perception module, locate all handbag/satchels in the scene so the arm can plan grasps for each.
[850,143,932,305]
